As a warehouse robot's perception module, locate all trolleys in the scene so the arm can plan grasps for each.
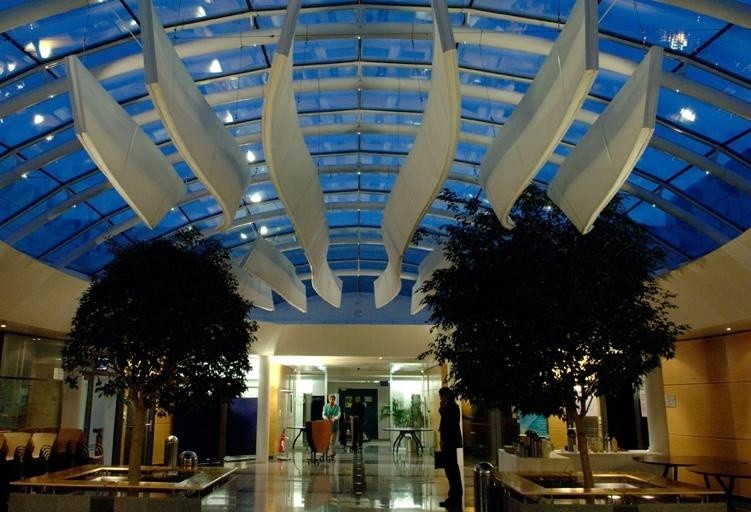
[306,419,336,466]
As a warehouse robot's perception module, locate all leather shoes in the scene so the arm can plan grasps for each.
[440,498,449,507]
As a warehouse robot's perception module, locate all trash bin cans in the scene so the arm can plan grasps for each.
[473,461,497,512]
[164,435,198,467]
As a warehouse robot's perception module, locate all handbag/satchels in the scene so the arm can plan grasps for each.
[434,451,444,469]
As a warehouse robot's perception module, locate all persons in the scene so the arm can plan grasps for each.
[338,394,369,453]
[321,394,342,462]
[437,386,463,512]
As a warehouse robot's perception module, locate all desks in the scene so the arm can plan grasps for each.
[383,425,434,455]
[287,425,310,448]
[632,453,751,494]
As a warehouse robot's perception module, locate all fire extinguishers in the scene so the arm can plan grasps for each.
[278,429,286,453]
[93,428,104,456]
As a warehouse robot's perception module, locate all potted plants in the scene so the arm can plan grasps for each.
[381,394,429,454]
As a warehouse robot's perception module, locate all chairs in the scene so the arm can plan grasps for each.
[3,427,104,478]
